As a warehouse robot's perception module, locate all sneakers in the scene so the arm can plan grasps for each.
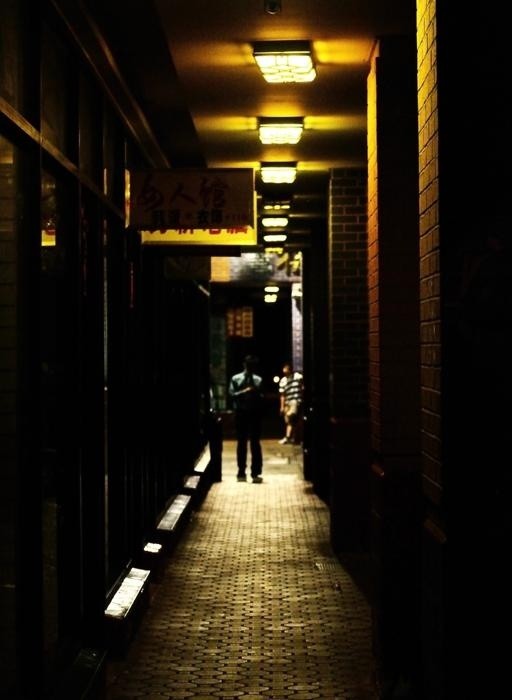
[278,437,292,444]
[237,469,258,477]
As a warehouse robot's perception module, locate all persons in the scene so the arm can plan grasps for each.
[278,364,304,445]
[226,353,264,484]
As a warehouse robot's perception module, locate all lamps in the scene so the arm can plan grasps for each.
[252,39,318,84]
[263,232,288,242]
[255,115,307,145]
[260,214,290,228]
[259,160,298,183]
